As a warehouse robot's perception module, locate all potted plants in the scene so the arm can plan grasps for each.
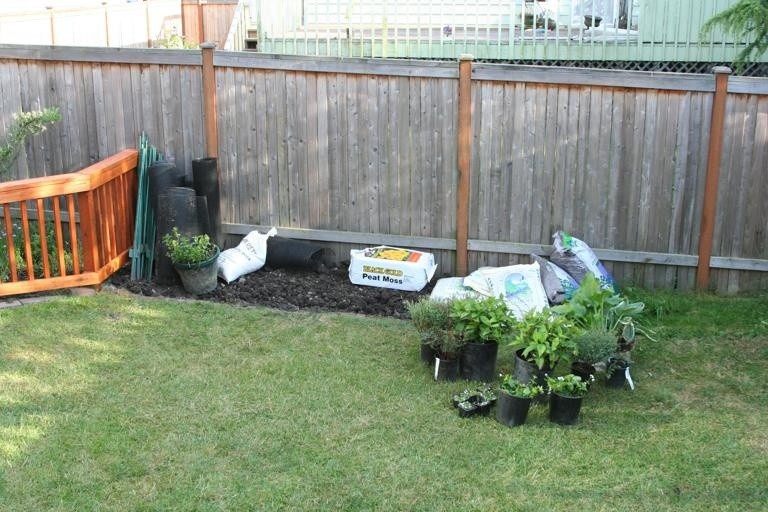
[162,229,222,298]
[406,269,649,427]
